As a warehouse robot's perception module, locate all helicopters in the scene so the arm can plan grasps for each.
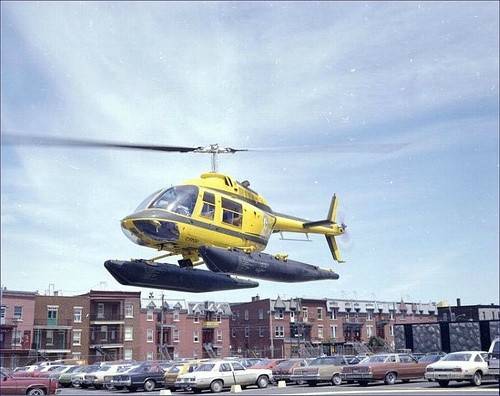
[0,134,408,293]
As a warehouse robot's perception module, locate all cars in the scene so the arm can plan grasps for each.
[0,339,500,396]
